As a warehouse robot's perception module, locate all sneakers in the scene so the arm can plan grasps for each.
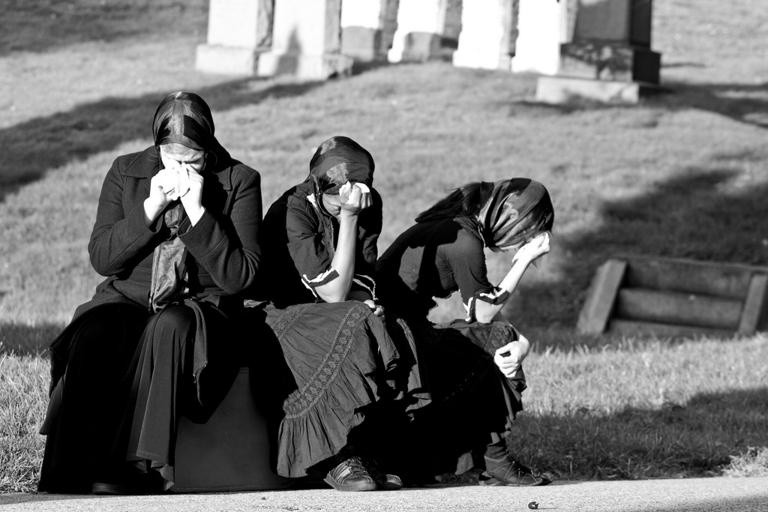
[378,471,402,491]
[483,452,543,486]
[323,455,378,491]
[93,473,175,494]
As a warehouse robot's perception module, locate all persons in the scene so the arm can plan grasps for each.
[377,177,556,488]
[32,87,292,496]
[242,135,436,493]
